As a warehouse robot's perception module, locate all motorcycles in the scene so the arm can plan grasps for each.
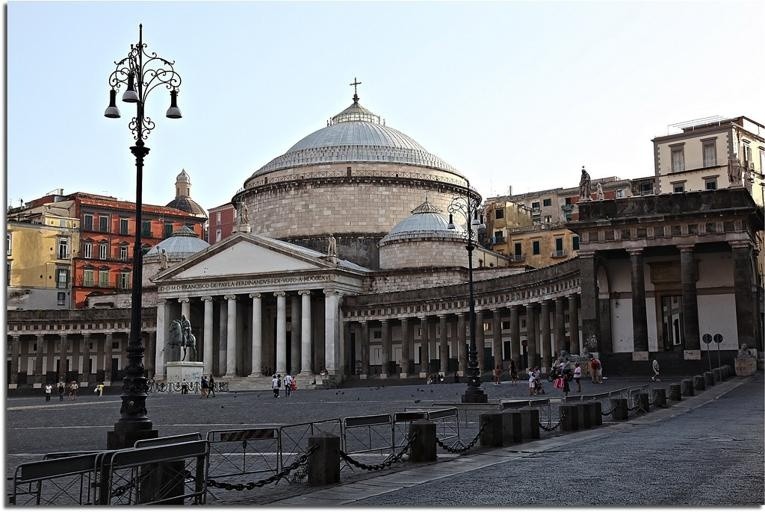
[546,365,574,382]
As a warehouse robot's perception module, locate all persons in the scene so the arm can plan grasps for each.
[181,374,216,399]
[509,361,518,386]
[494,365,502,384]
[44,380,105,402]
[240,202,249,224]
[328,233,336,256]
[527,355,603,396]
[578,169,591,199]
[271,371,297,398]
[492,368,496,379]
[651,356,661,382]
[430,374,444,383]
[160,248,169,268]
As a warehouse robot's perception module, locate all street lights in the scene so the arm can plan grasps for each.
[447,187,485,392]
[103,24,182,430]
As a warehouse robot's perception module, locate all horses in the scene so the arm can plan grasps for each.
[162,320,197,361]
